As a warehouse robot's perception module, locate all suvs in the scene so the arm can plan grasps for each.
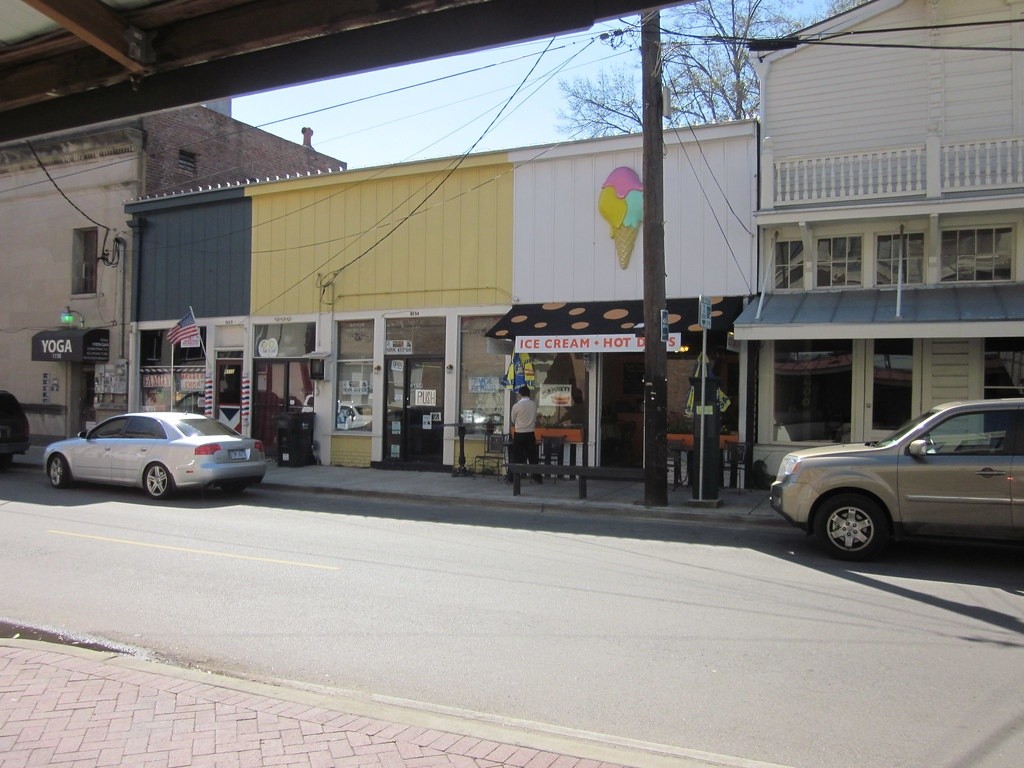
[769,399,1024,562]
[0,390,31,467]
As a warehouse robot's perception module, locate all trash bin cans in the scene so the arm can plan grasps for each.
[274,411,316,468]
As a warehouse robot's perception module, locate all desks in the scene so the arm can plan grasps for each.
[443,423,479,477]
[503,442,542,446]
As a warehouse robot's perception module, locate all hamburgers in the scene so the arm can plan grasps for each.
[551,391,569,404]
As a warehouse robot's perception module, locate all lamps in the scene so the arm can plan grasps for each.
[446,365,453,374]
[61,306,84,328]
[374,365,381,375]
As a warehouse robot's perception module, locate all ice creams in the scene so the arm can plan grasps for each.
[598,167,644,270]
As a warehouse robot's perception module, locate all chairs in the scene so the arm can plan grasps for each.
[474,433,510,482]
[530,435,567,484]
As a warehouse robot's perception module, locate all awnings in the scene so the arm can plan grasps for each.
[733,224,1024,340]
[31,328,110,363]
[483,293,757,354]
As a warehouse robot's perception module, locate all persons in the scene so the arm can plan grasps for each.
[503,386,543,486]
[558,387,588,428]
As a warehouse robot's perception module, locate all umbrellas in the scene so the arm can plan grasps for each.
[500,346,536,393]
[684,351,731,418]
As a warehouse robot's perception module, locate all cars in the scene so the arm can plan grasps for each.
[339,405,374,430]
[44,411,267,501]
[256,390,315,413]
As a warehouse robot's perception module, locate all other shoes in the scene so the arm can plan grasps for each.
[504,479,513,484]
[532,474,543,484]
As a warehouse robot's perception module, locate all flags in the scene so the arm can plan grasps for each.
[166,311,198,345]
